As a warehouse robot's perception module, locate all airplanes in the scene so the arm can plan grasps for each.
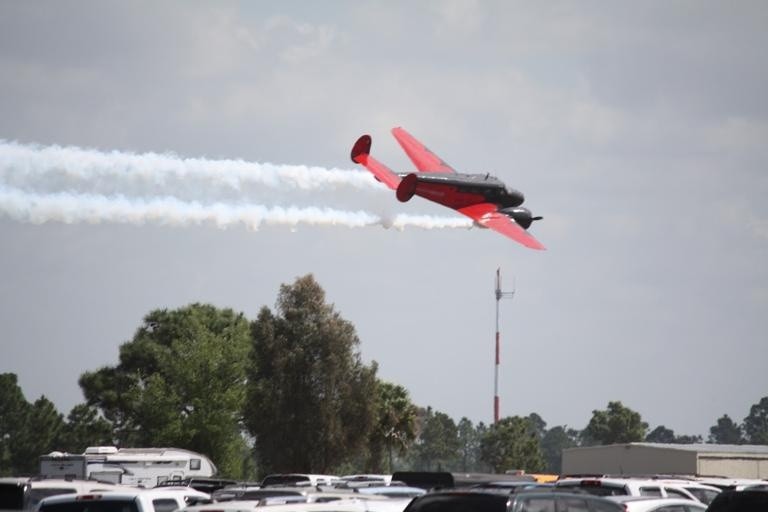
[352,126,545,250]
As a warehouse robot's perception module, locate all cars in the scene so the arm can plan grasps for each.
[0,447,767,511]
